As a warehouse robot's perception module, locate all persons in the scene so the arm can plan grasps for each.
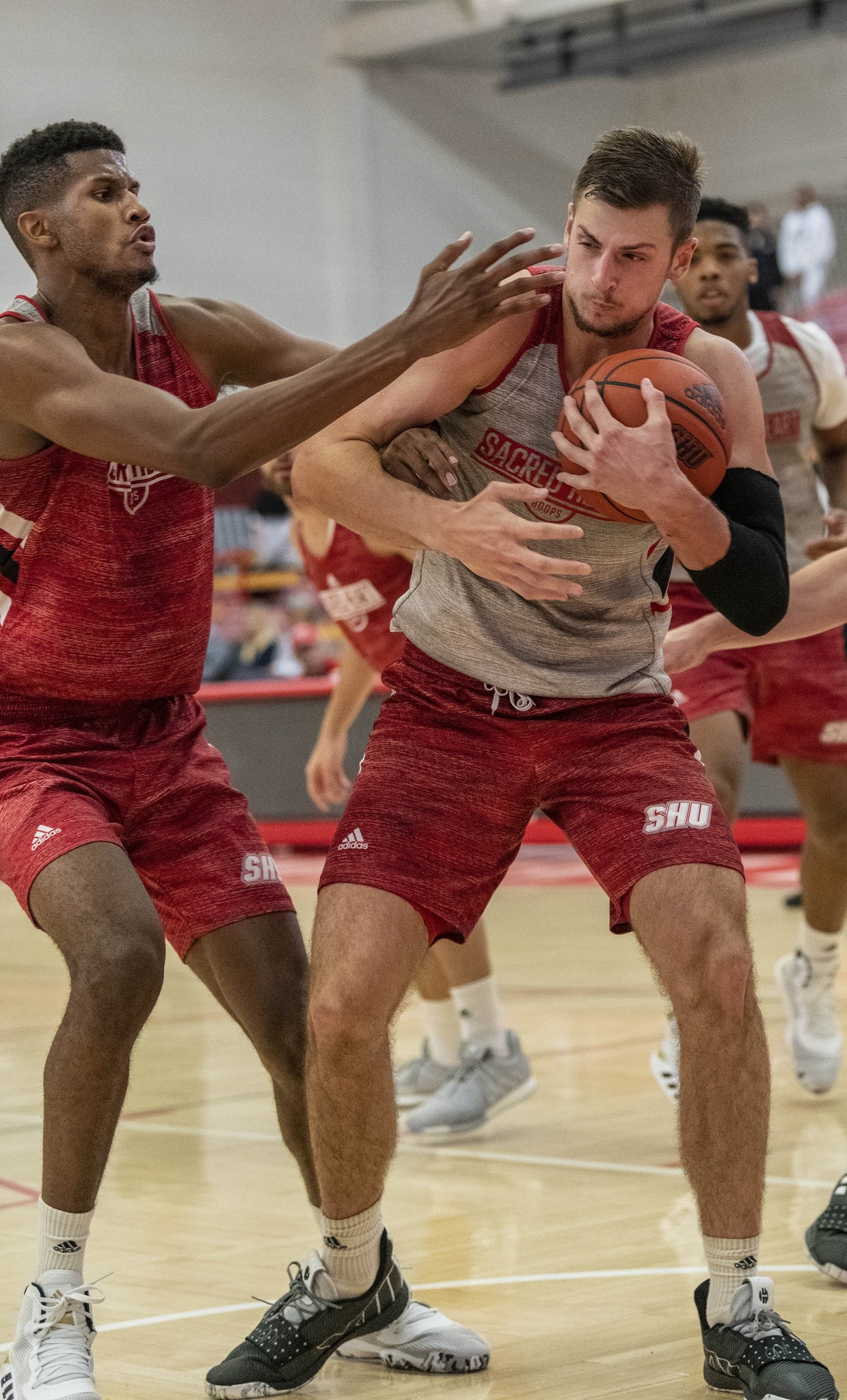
[247,186,847,1285]
[203,125,842,1400]
[203,129,787,1357]
[1,121,567,1400]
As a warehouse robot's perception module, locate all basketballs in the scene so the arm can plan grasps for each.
[556,349,732,524]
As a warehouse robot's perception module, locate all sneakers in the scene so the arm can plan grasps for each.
[392,1044,458,1105]
[407,1028,533,1137]
[335,1291,490,1373]
[691,1273,840,1400]
[806,1179,847,1281]
[206,1227,410,1398]
[778,951,840,1097]
[655,1023,682,1105]
[8,1275,111,1399]
